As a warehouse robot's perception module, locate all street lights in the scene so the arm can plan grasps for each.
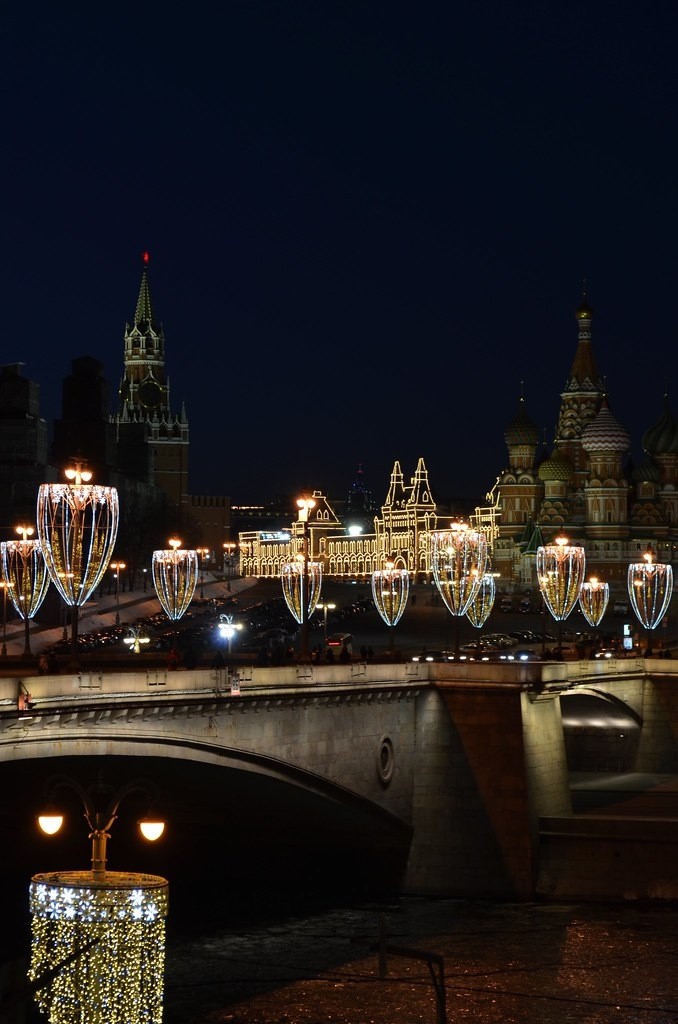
[26,774,170,1024]
[627,546,673,658]
[460,568,495,661]
[0,582,15,657]
[280,554,322,665]
[578,576,610,652]
[0,526,52,662]
[196,548,210,599]
[371,555,410,665]
[36,462,119,674]
[223,543,237,592]
[152,539,198,671]
[315,604,336,640]
[536,534,586,661]
[430,515,488,662]
[219,622,243,667]
[295,497,315,666]
[110,562,127,628]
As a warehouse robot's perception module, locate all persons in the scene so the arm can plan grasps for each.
[37,649,59,674]
[167,640,432,671]
[589,648,596,661]
[544,647,552,661]
[658,649,671,659]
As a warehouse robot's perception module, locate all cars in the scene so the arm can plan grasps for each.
[37,595,639,677]
